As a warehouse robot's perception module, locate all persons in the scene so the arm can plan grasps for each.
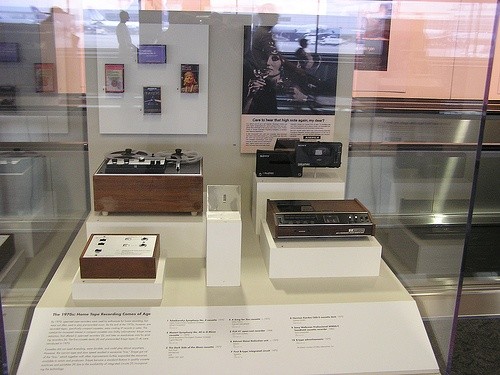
[249,3,326,115]
[182,72,198,93]
[114,10,140,60]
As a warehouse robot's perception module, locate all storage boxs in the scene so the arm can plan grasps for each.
[79,233,161,279]
